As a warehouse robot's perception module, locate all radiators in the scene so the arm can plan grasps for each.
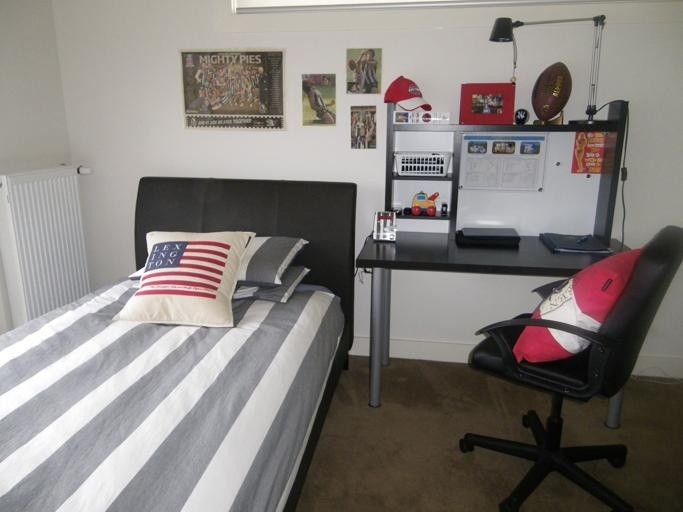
[1,163,94,328]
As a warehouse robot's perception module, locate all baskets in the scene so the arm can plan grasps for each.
[394,150,452,177]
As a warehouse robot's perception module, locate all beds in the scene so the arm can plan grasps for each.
[0,175,356,512]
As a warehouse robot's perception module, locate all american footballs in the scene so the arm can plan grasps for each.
[532,62,572,121]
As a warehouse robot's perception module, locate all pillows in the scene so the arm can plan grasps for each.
[507,247,641,365]
[107,223,311,332]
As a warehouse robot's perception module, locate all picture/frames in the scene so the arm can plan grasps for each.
[178,46,289,133]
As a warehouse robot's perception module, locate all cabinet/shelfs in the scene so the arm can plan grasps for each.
[385,98,633,244]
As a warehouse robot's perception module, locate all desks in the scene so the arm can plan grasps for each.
[357,229,635,430]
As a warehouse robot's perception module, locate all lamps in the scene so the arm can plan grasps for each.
[489,15,607,126]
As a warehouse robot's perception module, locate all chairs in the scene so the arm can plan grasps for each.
[454,224,683,510]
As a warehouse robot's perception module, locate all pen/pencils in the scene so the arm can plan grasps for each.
[577,236,588,244]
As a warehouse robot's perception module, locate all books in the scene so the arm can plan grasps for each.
[539,233,614,253]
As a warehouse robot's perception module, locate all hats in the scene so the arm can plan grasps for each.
[384,75,432,111]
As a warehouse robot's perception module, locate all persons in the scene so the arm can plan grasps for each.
[348,49,377,93]
[302,78,336,124]
[574,132,588,173]
